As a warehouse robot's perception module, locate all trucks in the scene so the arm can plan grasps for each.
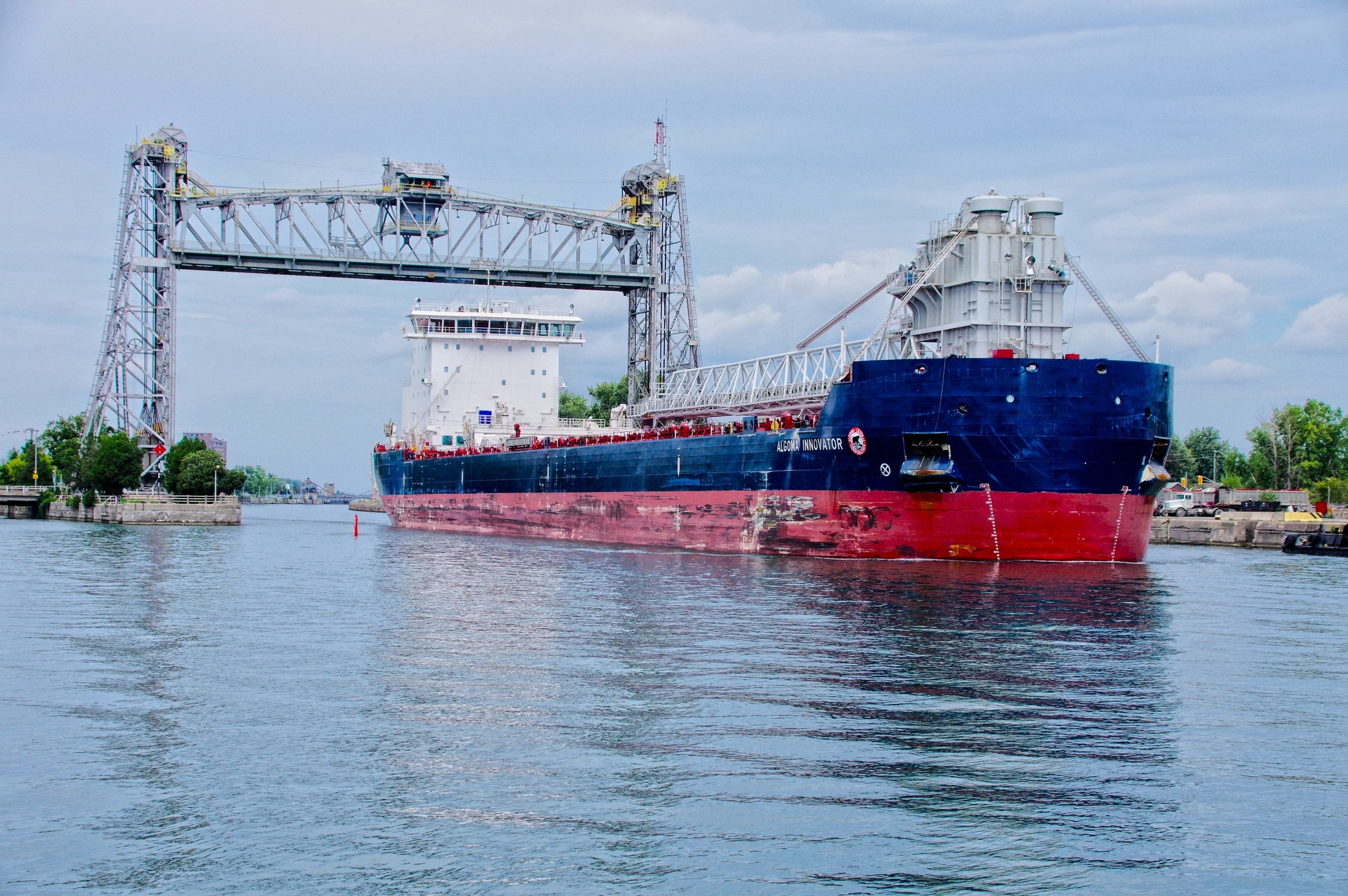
[1158,487,1312,517]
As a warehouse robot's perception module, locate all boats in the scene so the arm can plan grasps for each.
[369,187,1176,571]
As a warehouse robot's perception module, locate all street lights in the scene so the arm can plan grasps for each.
[214,465,220,502]
